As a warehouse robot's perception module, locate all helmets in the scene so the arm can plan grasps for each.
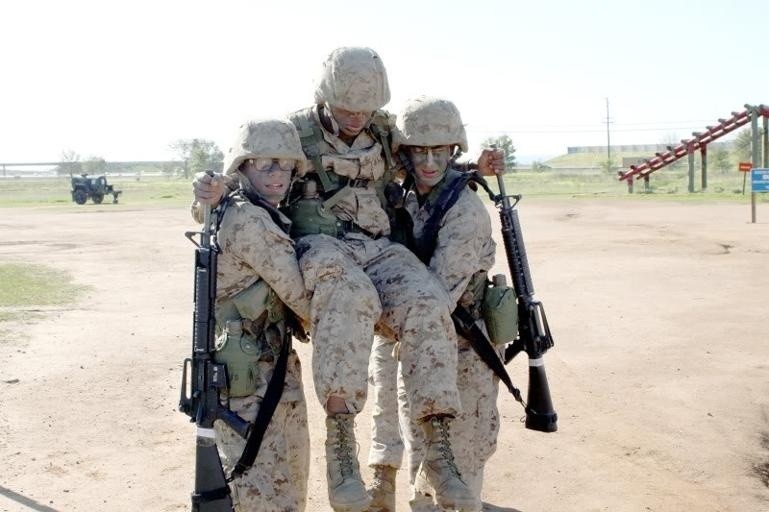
[314,46,391,114]
[222,117,307,176]
[391,96,468,153]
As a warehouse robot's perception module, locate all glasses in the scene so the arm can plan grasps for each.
[250,157,298,171]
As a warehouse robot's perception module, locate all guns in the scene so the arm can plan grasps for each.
[179,170,252,512]
[490,144,558,433]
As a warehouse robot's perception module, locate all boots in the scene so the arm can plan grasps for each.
[325,413,396,512]
[414,416,482,511]
